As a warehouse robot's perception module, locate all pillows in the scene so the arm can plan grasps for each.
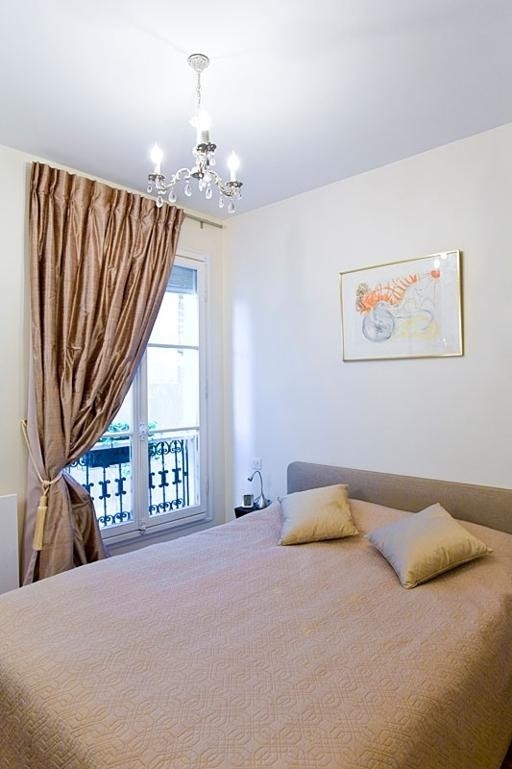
[362,501,494,589]
[277,482,358,546]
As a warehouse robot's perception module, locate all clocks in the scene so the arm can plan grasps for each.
[242,493,254,508]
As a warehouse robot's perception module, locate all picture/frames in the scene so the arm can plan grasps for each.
[339,250,465,362]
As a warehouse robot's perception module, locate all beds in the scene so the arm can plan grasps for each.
[0,461,510,769]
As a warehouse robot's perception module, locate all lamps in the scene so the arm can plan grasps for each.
[247,470,268,508]
[146,51,244,213]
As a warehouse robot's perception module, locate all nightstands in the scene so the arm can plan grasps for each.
[234,505,269,518]
